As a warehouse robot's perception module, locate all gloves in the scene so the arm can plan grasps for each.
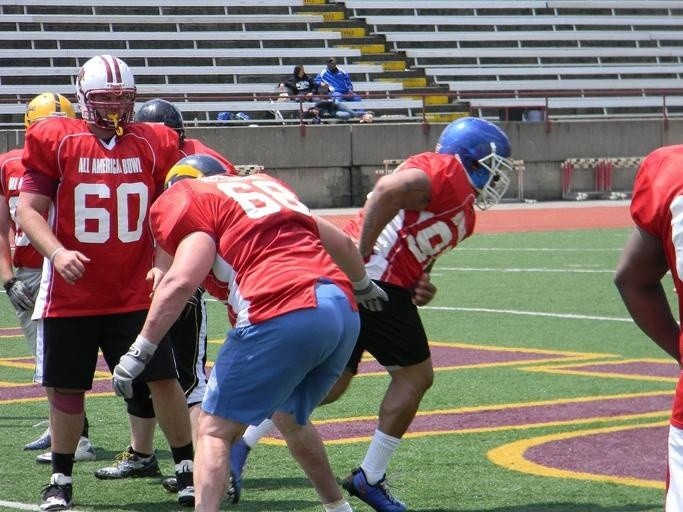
[112,336,159,400]
[179,287,206,318]
[5,279,35,314]
[351,274,390,312]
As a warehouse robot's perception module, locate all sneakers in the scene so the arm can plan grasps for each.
[23,427,51,450]
[39,483,74,511]
[180,470,241,506]
[164,474,189,490]
[94,454,160,477]
[342,466,406,511]
[39,437,94,462]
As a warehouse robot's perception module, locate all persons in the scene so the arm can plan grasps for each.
[0,92,96,464]
[93,98,238,493]
[279,64,321,102]
[308,101,374,119]
[222,117,514,512]
[314,57,361,102]
[614,145,683,512]
[13,54,196,511]
[112,154,389,512]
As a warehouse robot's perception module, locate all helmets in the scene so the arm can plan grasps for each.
[135,99,189,140]
[166,154,225,192]
[435,116,513,212]
[25,94,76,135]
[75,54,134,128]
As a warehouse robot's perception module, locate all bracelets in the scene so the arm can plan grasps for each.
[49,247,64,264]
